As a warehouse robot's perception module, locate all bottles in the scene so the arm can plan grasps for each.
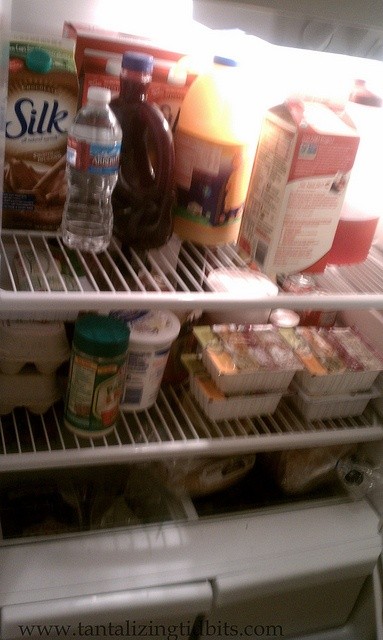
[171,56,254,246]
[59,87,124,253]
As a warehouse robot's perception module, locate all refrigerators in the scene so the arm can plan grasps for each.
[0,1,382,640]
